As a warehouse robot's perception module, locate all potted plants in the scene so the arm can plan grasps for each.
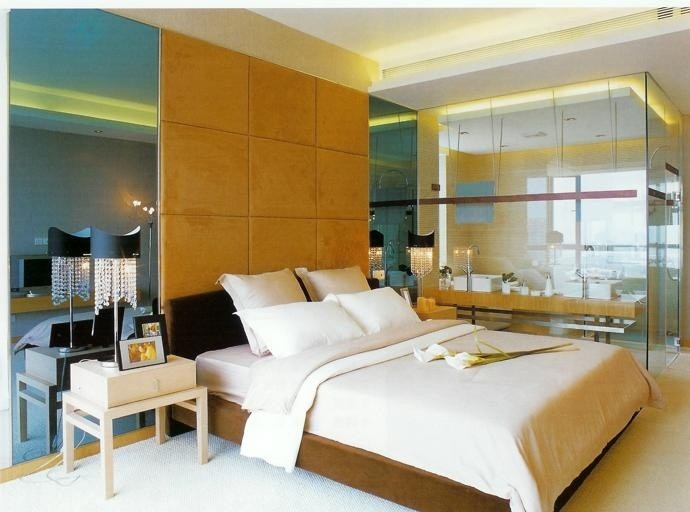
[438,265,453,290]
[501,270,517,294]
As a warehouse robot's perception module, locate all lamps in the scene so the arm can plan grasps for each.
[87,224,143,367]
[369,230,388,279]
[408,230,436,301]
[48,223,94,353]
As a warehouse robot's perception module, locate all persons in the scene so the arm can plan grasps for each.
[138,346,150,361]
[144,343,157,361]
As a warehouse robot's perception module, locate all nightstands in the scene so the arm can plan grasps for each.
[412,302,460,321]
[16,340,113,456]
[63,356,212,497]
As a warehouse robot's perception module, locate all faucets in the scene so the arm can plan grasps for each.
[462,244,481,292]
[575,244,595,300]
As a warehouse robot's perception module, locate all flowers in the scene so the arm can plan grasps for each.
[411,327,581,372]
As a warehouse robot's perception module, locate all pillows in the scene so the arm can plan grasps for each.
[295,266,370,302]
[232,293,365,358]
[216,267,310,358]
[337,283,421,336]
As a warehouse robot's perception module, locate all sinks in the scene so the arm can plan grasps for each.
[562,278,624,300]
[453,273,503,293]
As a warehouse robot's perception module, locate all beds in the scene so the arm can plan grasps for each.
[166,278,667,511]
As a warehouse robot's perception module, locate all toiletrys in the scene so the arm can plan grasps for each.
[531,290,541,296]
[520,282,529,295]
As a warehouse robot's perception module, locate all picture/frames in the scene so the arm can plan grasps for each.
[47,321,92,347]
[116,335,168,371]
[398,285,412,304]
[94,307,126,345]
[134,313,170,356]
